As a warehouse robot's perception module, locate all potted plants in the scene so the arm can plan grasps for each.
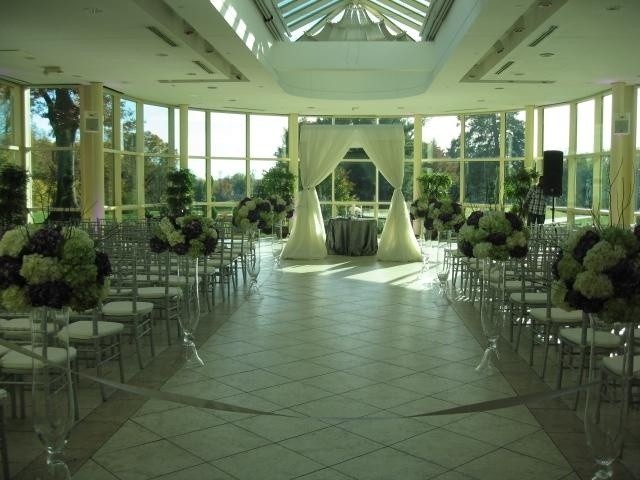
[410,173,452,241]
[255,161,296,239]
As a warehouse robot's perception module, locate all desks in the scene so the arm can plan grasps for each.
[329,216,377,256]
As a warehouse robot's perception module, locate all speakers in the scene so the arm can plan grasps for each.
[83,110,100,133]
[613,112,631,135]
[542,150,563,197]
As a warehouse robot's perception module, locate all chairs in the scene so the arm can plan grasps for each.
[423,228,455,264]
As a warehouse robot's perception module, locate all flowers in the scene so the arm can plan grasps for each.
[231,197,275,230]
